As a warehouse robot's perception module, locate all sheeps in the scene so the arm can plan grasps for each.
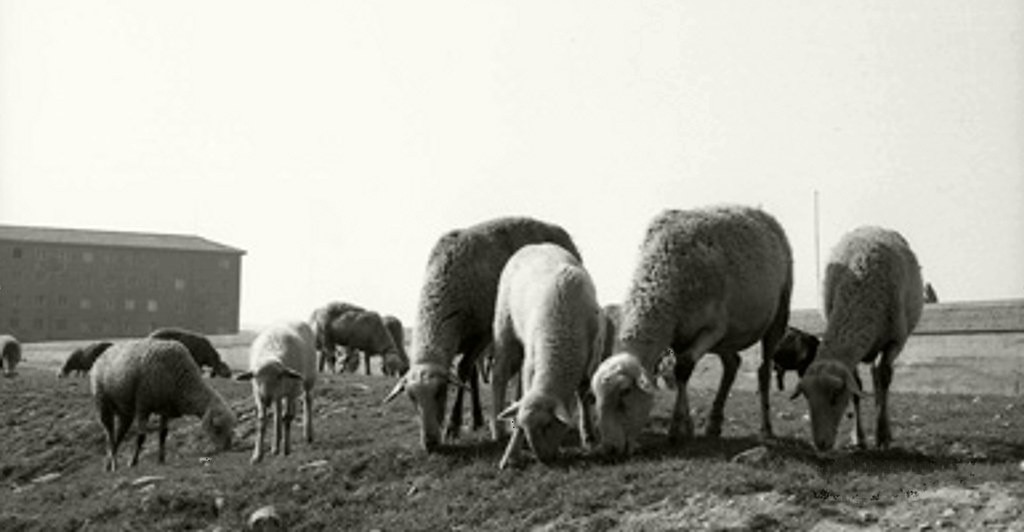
[148,327,232,379]
[57,342,113,379]
[380,205,926,471]
[236,322,318,465]
[90,339,239,473]
[309,301,410,378]
[0,334,21,378]
